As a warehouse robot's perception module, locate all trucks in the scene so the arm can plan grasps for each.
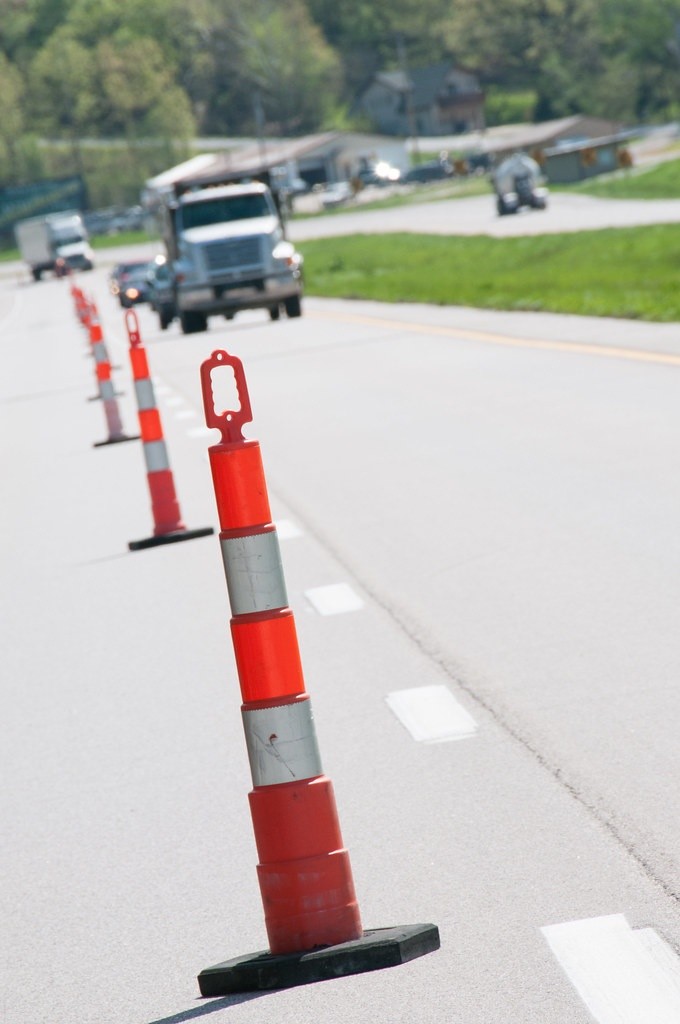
[489,152,550,215]
[12,208,97,282]
[146,153,302,335]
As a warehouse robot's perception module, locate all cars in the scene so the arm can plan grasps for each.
[112,260,161,309]
[147,261,178,329]
[405,159,455,184]
[361,161,403,187]
